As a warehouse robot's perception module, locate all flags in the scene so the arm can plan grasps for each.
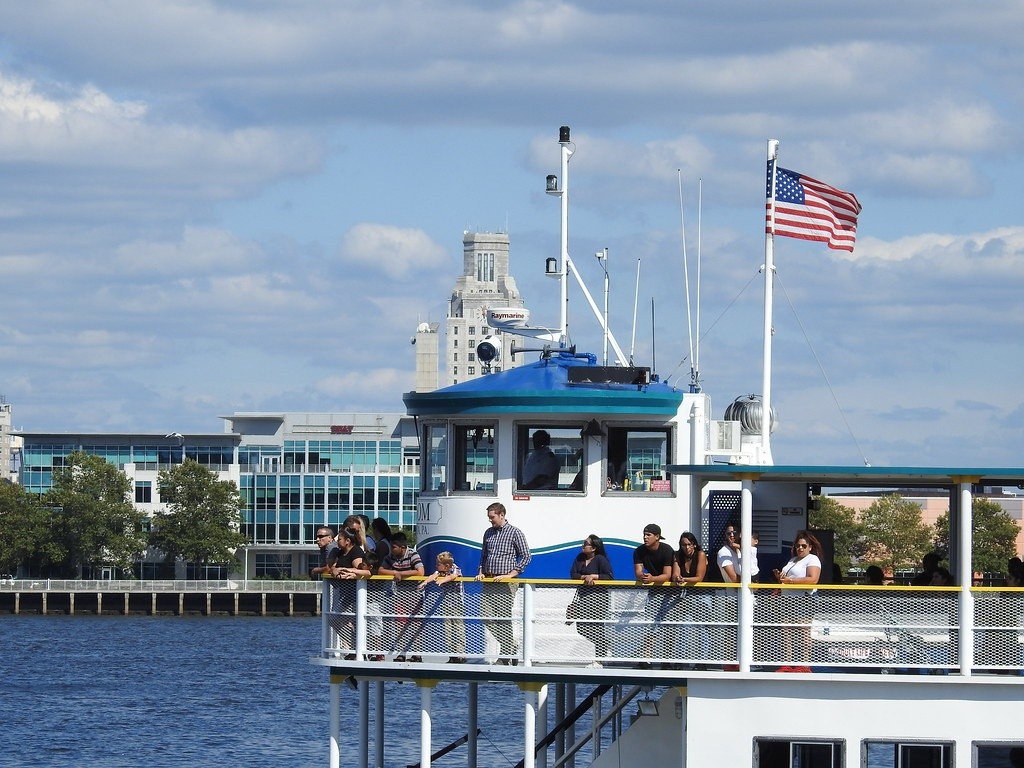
[765,158,862,254]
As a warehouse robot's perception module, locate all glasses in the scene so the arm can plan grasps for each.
[725,531,737,537]
[584,541,592,546]
[795,544,807,549]
[316,535,329,539]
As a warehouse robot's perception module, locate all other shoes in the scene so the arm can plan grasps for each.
[633,662,653,670]
[661,663,672,670]
[493,658,509,665]
[446,657,466,662]
[512,645,518,666]
[775,666,811,672]
[692,664,707,670]
[393,655,422,662]
[345,654,386,661]
[601,651,612,664]
[585,662,603,670]
[724,665,739,671]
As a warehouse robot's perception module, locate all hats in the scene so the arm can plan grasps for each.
[644,524,665,540]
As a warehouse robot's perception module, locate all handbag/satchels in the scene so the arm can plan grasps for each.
[566,589,580,625]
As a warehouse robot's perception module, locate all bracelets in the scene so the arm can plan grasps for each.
[788,578,793,584]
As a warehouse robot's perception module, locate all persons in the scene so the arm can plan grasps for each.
[773,532,821,672]
[417,552,467,663]
[570,534,616,669]
[909,554,954,587]
[312,514,424,662]
[633,524,712,671]
[475,501,532,666]
[717,523,760,671]
[522,430,561,490]
[1008,557,1024,586]
[881,564,894,586]
[865,566,883,585]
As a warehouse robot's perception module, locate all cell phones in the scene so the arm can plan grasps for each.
[773,569,780,575]
